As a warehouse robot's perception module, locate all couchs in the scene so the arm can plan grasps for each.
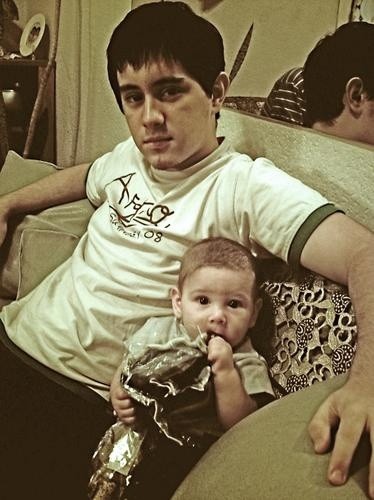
[1,105,373,500]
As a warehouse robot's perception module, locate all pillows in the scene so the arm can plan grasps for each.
[0,147,98,299]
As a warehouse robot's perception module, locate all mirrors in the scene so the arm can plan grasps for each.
[132,1,373,146]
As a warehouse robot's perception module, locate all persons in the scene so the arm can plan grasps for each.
[259,61,308,127]
[297,21,372,146]
[88,241,277,500]
[1,0,373,500]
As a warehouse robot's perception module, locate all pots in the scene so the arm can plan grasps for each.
[2,87,29,111]
